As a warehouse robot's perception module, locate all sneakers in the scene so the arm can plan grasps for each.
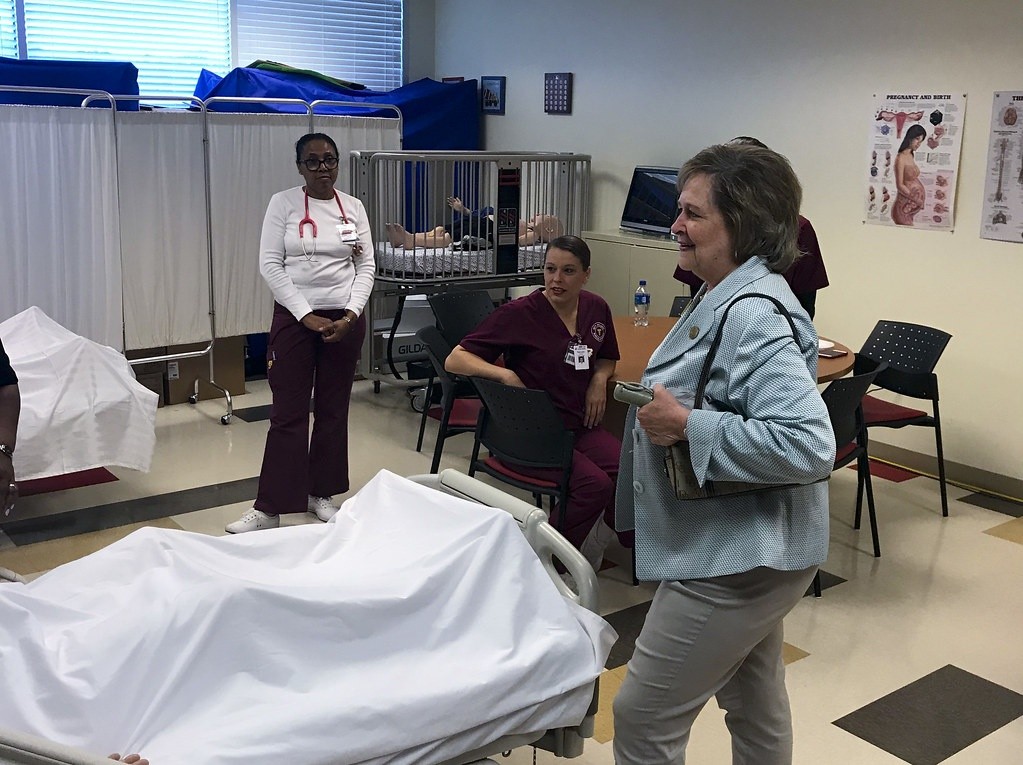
[225,507,279,534]
[303,495,339,522]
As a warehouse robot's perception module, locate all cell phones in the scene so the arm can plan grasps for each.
[818,350,848,359]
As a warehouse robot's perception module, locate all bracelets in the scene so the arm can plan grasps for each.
[683,429,688,438]
[343,316,350,322]
[0,444,12,458]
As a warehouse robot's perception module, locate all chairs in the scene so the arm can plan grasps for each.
[853,320,952,529]
[415,288,499,475]
[469,373,565,522]
[806,360,891,598]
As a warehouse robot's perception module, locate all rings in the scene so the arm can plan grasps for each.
[10,484,14,486]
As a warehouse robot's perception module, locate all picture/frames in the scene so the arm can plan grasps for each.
[442,76,464,84]
[482,76,506,115]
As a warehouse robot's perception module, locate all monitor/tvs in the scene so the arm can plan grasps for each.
[620,164,685,241]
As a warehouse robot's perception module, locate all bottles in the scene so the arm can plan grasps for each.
[634,280,650,326]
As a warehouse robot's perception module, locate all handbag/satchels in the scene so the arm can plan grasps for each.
[663,289,833,502]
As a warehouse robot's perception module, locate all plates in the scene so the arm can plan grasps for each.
[819,339,834,351]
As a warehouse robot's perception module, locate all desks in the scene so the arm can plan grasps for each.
[601,315,856,440]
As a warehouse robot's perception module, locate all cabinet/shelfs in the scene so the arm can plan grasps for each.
[582,230,692,317]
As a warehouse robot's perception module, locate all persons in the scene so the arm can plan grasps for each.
[445,236,636,596]
[0,337,20,516]
[225,133,375,534]
[673,136,829,322]
[579,357,584,364]
[891,125,927,225]
[613,141,836,765]
[385,197,563,250]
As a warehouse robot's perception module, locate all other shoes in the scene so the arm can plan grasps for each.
[552,553,567,575]
[579,515,615,573]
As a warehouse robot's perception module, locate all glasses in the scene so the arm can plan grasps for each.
[299,157,339,172]
